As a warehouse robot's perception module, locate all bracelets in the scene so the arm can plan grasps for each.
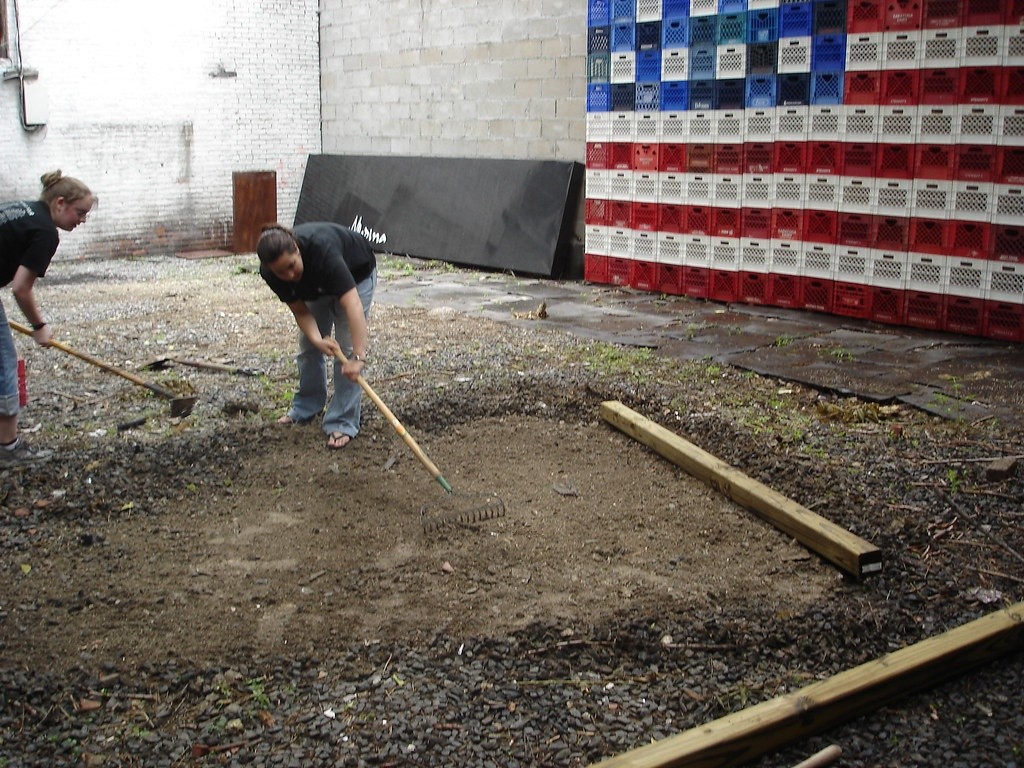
[32,322,47,330]
[352,354,366,362]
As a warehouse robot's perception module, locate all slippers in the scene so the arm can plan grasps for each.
[326,432,351,450]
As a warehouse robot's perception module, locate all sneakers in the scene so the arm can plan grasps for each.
[0,438,52,469]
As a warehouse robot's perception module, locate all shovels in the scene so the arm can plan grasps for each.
[135,356,265,379]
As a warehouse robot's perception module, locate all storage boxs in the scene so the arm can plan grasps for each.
[581,0,1024,342]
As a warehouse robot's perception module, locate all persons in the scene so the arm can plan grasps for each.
[257,220,377,449]
[0,169,98,451]
